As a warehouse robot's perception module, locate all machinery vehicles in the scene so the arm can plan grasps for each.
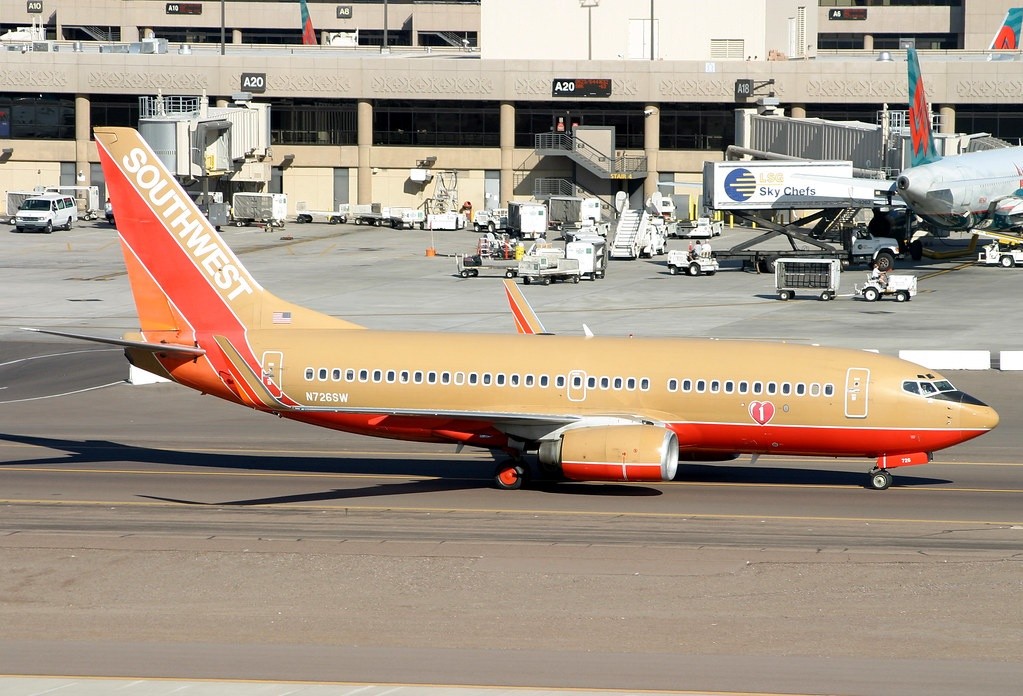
[977,241,1023,268]
[426,210,467,231]
[565,238,606,283]
[472,209,508,233]
[610,194,668,260]
[673,218,724,238]
[667,250,719,278]
[852,274,919,301]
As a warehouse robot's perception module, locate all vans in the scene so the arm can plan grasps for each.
[16,194,77,234]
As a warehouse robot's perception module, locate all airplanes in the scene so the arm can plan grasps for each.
[18,124,1002,490]
[894,145,1022,261]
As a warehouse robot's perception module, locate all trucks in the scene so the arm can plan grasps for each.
[714,223,900,271]
[506,201,549,242]
